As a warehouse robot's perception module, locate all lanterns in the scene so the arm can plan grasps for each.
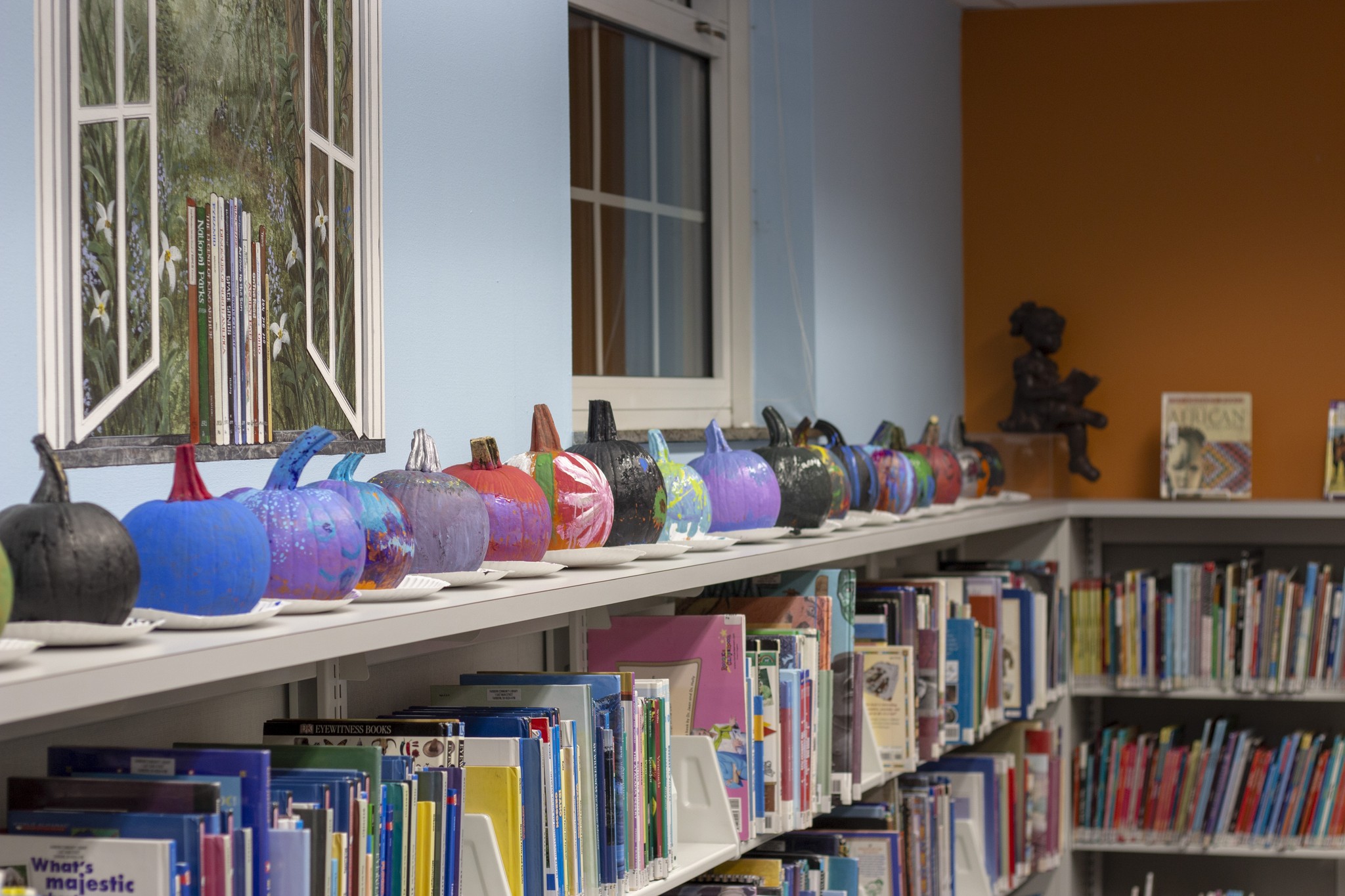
[221,426,369,599]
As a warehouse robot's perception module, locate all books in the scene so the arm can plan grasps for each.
[1,555,1066,896]
[1065,546,1345,896]
[1160,393,1252,500]
[1324,399,1344,500]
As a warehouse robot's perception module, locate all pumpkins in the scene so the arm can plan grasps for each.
[0,401,1001,642]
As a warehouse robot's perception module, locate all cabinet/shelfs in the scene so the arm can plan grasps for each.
[0,498,1066,896]
[1068,497,1345,896]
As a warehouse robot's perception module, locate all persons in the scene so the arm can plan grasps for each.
[994,300,1110,479]
